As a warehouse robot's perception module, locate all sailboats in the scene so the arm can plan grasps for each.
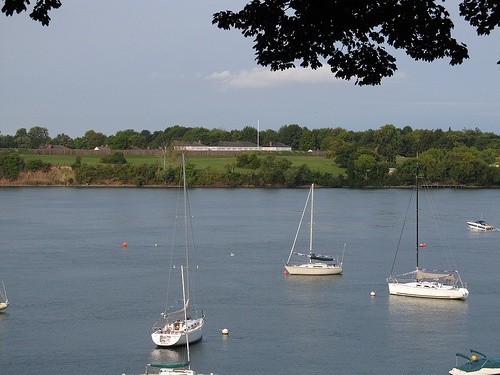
[150,150,206,347]
[388,152,469,300]
[466,211,497,234]
[283,177,347,276]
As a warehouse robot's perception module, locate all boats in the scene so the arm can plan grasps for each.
[449,342,500,375]
[122,349,212,375]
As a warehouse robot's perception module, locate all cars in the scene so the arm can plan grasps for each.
[308,149,313,153]
